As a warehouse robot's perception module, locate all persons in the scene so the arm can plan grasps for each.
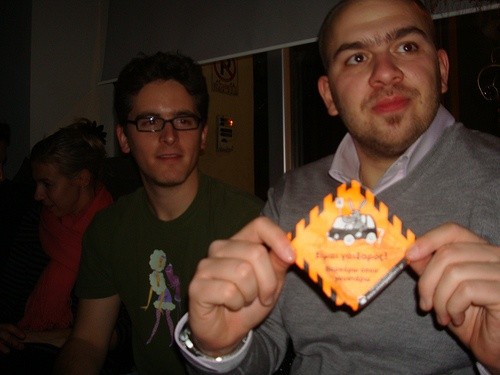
[40,48,252,375]
[163,0,499,375]
[0,118,132,375]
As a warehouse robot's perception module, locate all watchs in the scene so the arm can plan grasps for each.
[178,321,249,358]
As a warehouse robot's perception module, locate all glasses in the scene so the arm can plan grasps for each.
[126,116,201,133]
